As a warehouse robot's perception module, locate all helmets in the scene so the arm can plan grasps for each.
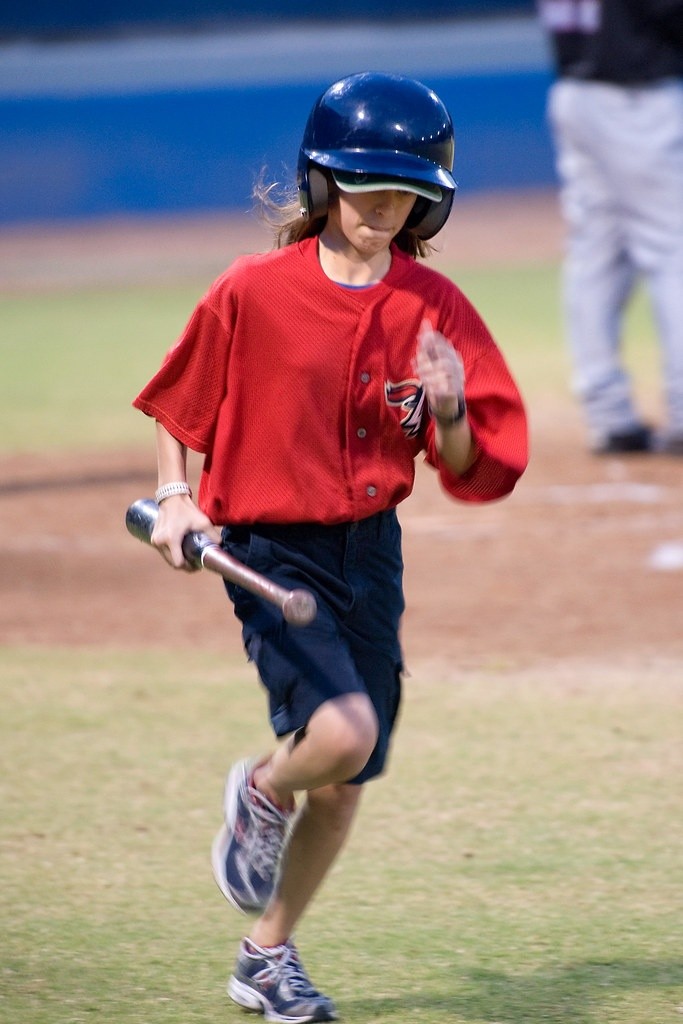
[297,71,458,241]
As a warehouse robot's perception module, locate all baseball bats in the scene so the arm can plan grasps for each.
[125,497,317,628]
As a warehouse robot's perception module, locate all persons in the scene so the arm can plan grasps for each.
[133,71,528,1024]
[537,1,682,451]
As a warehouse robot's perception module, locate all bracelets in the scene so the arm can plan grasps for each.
[156,483,192,504]
[427,394,465,425]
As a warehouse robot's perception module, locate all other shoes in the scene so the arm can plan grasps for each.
[663,428,683,454]
[595,420,654,453]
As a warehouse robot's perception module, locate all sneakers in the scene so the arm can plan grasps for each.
[226,935,339,1024]
[209,749,298,921]
